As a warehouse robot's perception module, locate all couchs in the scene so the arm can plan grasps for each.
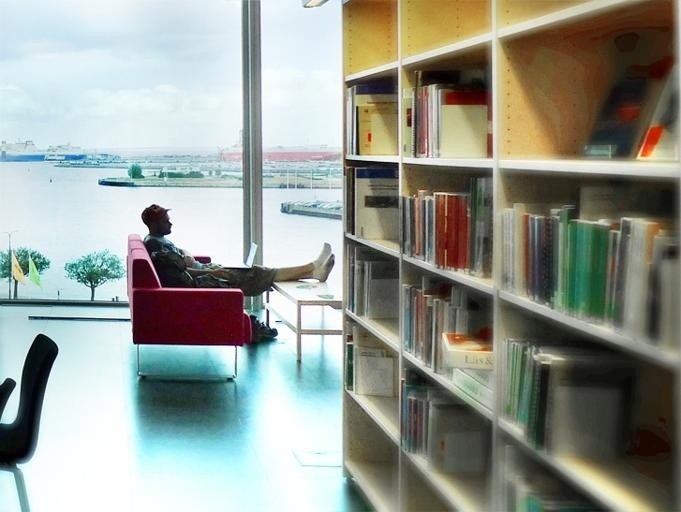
[125,231,252,383]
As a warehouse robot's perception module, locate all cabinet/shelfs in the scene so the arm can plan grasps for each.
[337,0,680,512]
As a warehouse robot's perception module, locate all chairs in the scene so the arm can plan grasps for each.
[0,377,18,416]
[1,331,59,511]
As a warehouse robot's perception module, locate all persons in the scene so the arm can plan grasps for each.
[139,203,336,297]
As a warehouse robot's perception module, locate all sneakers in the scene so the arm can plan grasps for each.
[249,315,277,342]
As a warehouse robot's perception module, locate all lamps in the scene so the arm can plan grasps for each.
[300,0,329,8]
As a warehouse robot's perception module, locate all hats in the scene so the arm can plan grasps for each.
[140,204,170,224]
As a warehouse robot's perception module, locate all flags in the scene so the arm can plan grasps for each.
[29,254,43,289]
[12,251,26,286]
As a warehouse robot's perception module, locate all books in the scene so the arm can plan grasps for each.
[343,82,397,157]
[402,70,493,158]
[342,165,680,512]
[583,61,680,160]
